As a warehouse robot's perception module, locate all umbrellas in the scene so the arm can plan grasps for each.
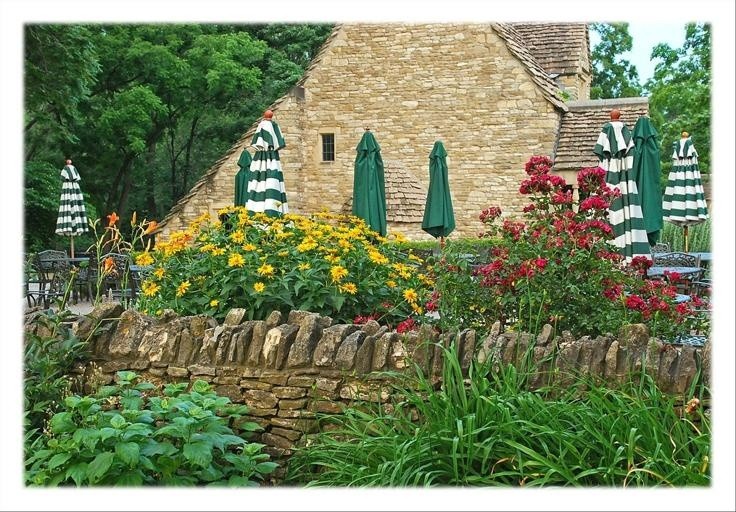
[234,148,252,207]
[662,131,709,254]
[55,160,90,258]
[245,111,289,215]
[630,110,663,246]
[352,128,387,238]
[421,140,456,252]
[593,108,652,266]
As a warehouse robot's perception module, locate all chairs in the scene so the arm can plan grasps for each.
[649,243,713,350]
[24,249,155,310]
